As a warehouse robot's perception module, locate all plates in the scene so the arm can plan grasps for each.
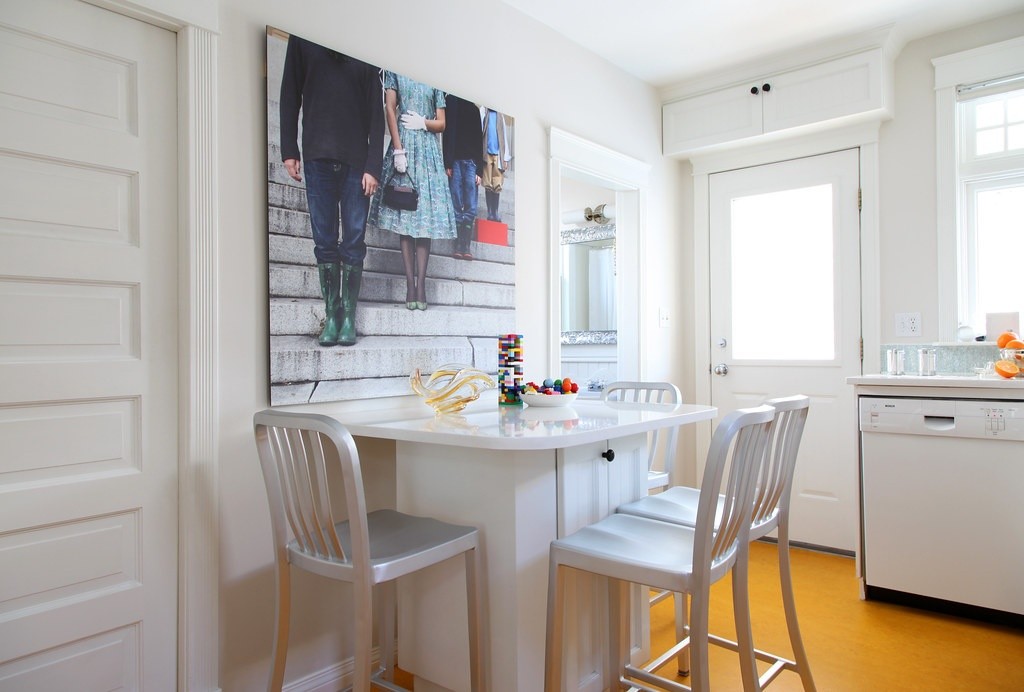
[518,391,578,407]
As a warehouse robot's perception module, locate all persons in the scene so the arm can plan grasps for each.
[368,71,457,311]
[279,34,386,347]
[441,92,484,261]
[478,106,514,222]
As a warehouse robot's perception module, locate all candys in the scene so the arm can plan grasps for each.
[523,378,578,395]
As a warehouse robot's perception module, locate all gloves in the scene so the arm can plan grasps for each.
[401,110,429,132]
[393,148,408,173]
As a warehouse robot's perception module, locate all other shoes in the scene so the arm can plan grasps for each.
[406,299,416,310]
[417,301,427,311]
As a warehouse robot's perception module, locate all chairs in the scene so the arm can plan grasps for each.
[547,403,777,691]
[251,407,486,691]
[614,393,821,692]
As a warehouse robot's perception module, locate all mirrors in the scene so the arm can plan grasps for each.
[557,224,617,347]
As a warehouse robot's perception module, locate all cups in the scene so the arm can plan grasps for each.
[917,348,937,376]
[885,349,905,375]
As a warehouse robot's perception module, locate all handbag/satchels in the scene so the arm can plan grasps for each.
[382,168,419,213]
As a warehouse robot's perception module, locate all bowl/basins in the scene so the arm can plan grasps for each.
[1000,347,1024,378]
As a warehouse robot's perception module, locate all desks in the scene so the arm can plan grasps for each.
[331,402,720,692]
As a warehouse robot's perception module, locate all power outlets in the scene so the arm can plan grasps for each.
[895,311,922,337]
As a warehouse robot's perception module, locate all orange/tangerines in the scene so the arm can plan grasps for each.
[1004,341,1024,349]
[995,361,1019,377]
[998,332,1017,348]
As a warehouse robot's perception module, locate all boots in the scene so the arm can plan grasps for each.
[494,192,501,222]
[463,223,473,260]
[454,222,464,258]
[336,263,363,345]
[485,190,495,220]
[318,262,341,346]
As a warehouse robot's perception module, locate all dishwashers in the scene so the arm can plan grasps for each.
[858,398,1024,618]
